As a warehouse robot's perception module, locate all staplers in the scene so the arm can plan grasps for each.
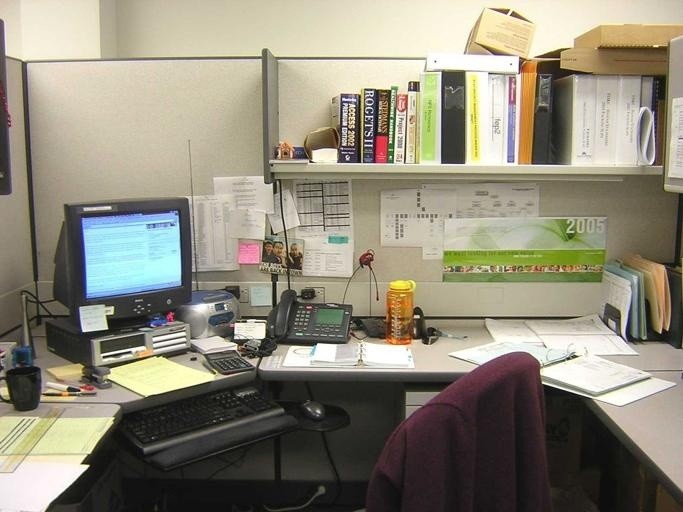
[82,366,112,389]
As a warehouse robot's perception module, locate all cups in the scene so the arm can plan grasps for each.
[0,365,41,412]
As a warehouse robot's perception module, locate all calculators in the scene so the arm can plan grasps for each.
[204,349,255,375]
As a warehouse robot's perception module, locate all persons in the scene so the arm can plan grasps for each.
[286,243,303,269]
[263,241,273,263]
[270,241,284,264]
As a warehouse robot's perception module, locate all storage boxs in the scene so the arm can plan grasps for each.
[464,6,536,63]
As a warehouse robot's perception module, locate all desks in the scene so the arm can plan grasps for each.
[0,318,683,512]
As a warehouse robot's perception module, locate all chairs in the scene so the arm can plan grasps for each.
[363,356,554,511]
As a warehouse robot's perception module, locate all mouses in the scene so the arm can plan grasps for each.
[300,399,327,422]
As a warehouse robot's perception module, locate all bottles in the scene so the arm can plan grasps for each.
[385,278,416,345]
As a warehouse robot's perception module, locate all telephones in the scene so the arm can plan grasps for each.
[274,290,352,346]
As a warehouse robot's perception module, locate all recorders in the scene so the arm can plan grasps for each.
[175,289,241,339]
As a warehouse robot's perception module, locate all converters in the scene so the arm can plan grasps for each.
[225,286,240,299]
[301,289,315,300]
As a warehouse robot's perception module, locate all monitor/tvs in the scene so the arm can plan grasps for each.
[53,197,192,331]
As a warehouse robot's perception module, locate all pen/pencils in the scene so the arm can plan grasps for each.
[203,362,216,375]
[42,382,97,395]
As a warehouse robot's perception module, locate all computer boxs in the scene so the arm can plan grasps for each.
[45,317,192,369]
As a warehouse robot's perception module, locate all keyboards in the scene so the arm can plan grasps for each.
[118,385,285,457]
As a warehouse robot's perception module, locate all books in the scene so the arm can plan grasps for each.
[282,343,321,369]
[313,341,418,371]
[330,71,668,170]
[448,338,652,396]
